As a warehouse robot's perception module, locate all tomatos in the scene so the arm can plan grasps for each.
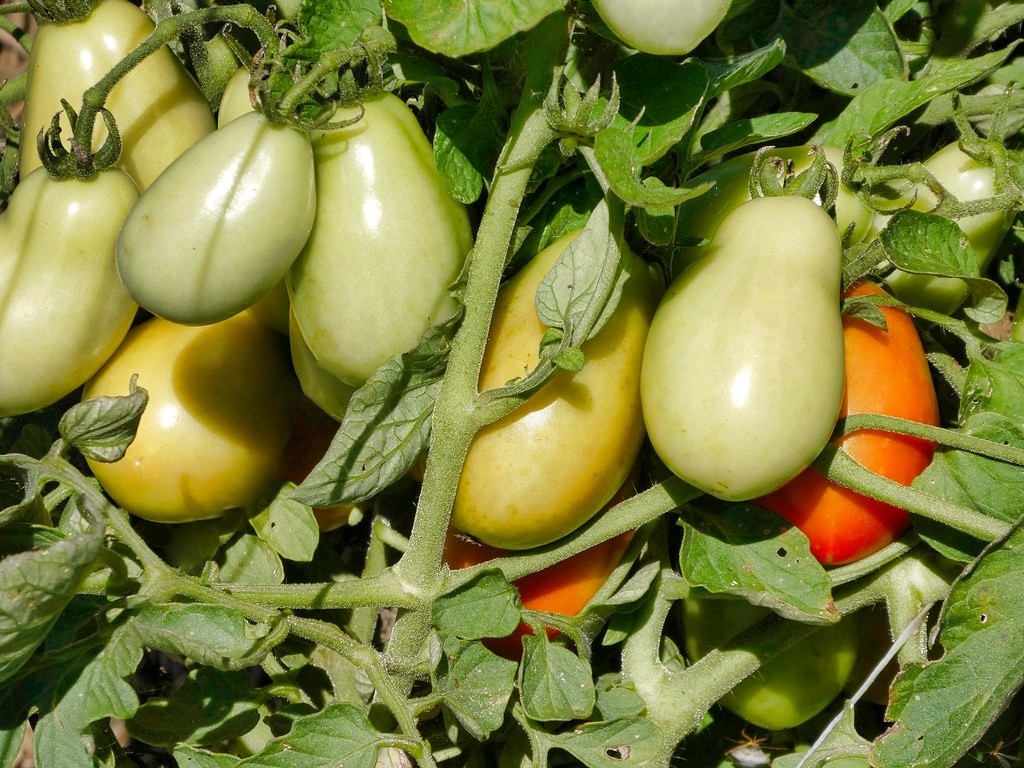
[0,0,1024,728]
